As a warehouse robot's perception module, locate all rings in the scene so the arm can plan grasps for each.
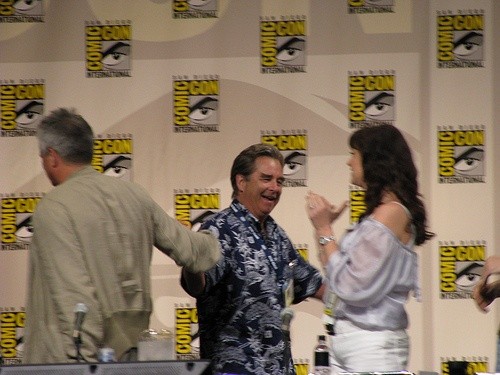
[309,206,315,209]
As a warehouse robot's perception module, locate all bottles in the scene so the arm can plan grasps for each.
[313,335,331,375]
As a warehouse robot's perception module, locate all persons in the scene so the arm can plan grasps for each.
[180,143,328,375]
[305,123,436,375]
[22,108,221,366]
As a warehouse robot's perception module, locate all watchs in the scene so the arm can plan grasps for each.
[318,235,335,245]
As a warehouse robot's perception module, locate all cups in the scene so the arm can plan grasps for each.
[156,329,174,362]
[137,328,157,361]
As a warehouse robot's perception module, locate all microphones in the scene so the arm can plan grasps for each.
[73,303,88,343]
[281,308,294,333]
